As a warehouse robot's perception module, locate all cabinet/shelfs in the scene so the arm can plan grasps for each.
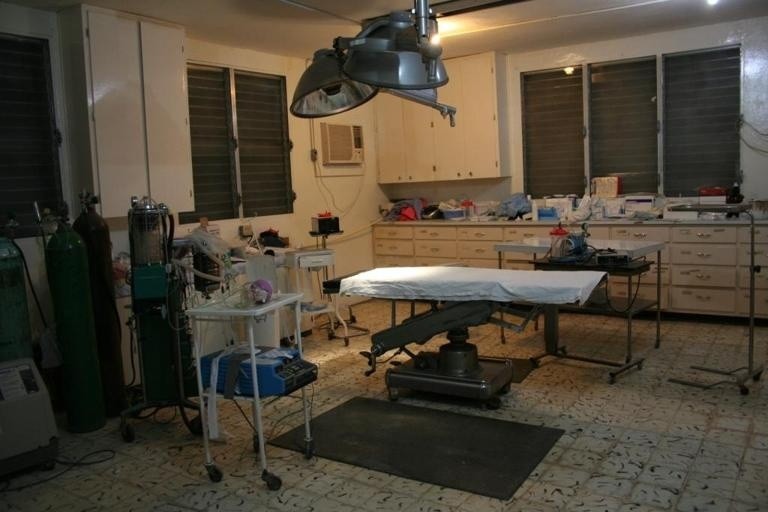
[375,51,498,184]
[187,291,309,490]
[115,275,247,386]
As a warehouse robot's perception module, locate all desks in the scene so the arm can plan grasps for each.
[496,237,666,383]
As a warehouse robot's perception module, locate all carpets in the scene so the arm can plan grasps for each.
[264,397,565,501]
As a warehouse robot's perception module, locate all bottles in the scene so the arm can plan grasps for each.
[532,200,538,221]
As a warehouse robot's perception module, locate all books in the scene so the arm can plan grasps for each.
[698,196,726,205]
[589,176,624,197]
[697,185,728,195]
[592,190,658,219]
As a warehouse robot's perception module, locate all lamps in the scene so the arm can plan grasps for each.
[291,0,456,127]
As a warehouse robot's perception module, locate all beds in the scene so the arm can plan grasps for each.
[342,268,608,405]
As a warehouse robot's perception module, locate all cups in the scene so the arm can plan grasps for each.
[550,229,568,258]
